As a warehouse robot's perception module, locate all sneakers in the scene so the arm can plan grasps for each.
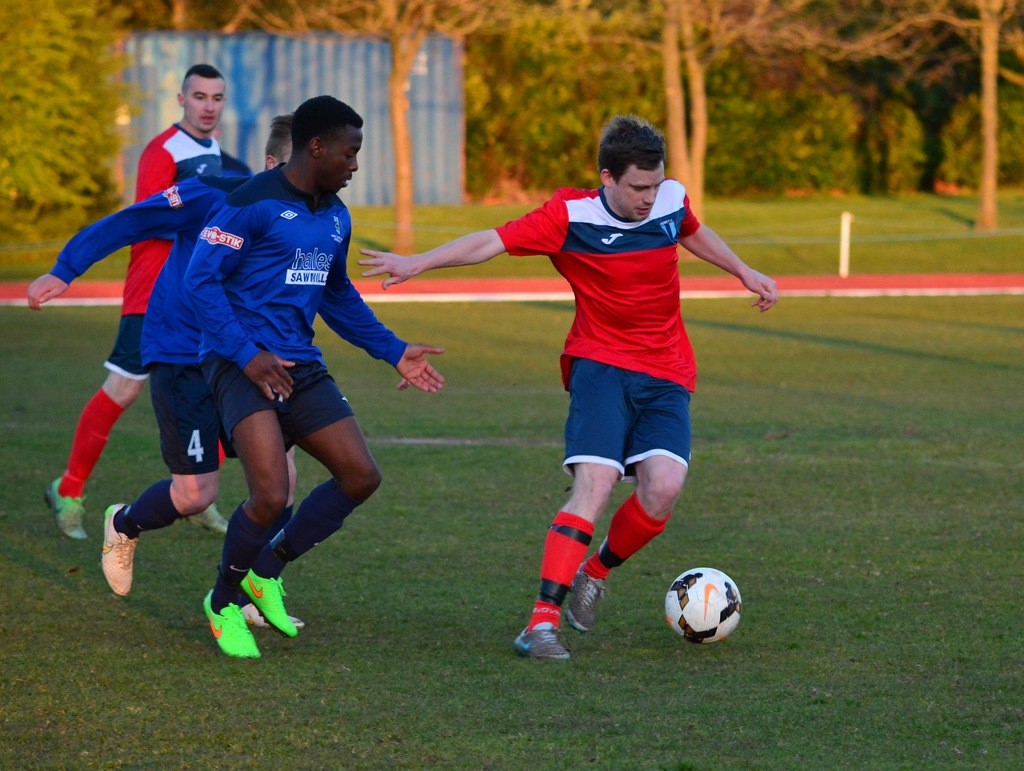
[561,559,604,631]
[515,621,571,659]
[204,589,262,658]
[237,568,298,636]
[44,476,88,539]
[241,602,305,628]
[102,503,140,596]
[188,501,228,535]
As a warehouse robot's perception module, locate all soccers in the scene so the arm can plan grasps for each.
[664,566,743,644]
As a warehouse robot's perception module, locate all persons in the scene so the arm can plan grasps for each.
[44,64,229,540]
[184,96,444,657]
[360,113,778,658]
[28,112,413,629]
